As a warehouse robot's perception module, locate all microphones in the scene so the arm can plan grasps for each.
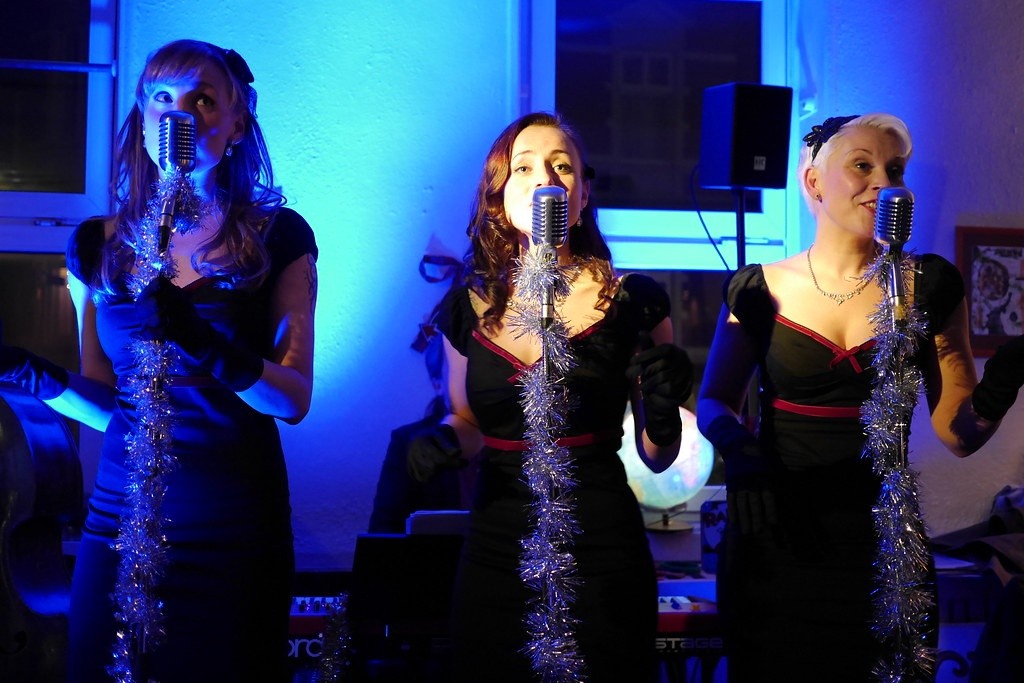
[533,186,568,328]
[873,185,913,327]
[156,110,197,256]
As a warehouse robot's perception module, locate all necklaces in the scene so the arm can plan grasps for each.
[506,258,582,309]
[807,244,875,304]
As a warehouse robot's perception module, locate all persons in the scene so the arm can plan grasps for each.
[407,111,694,683]
[0,39,318,683]
[697,114,1024,683]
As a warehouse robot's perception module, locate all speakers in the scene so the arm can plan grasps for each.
[699,81,793,187]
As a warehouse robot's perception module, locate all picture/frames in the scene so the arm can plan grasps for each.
[955,225,1024,359]
